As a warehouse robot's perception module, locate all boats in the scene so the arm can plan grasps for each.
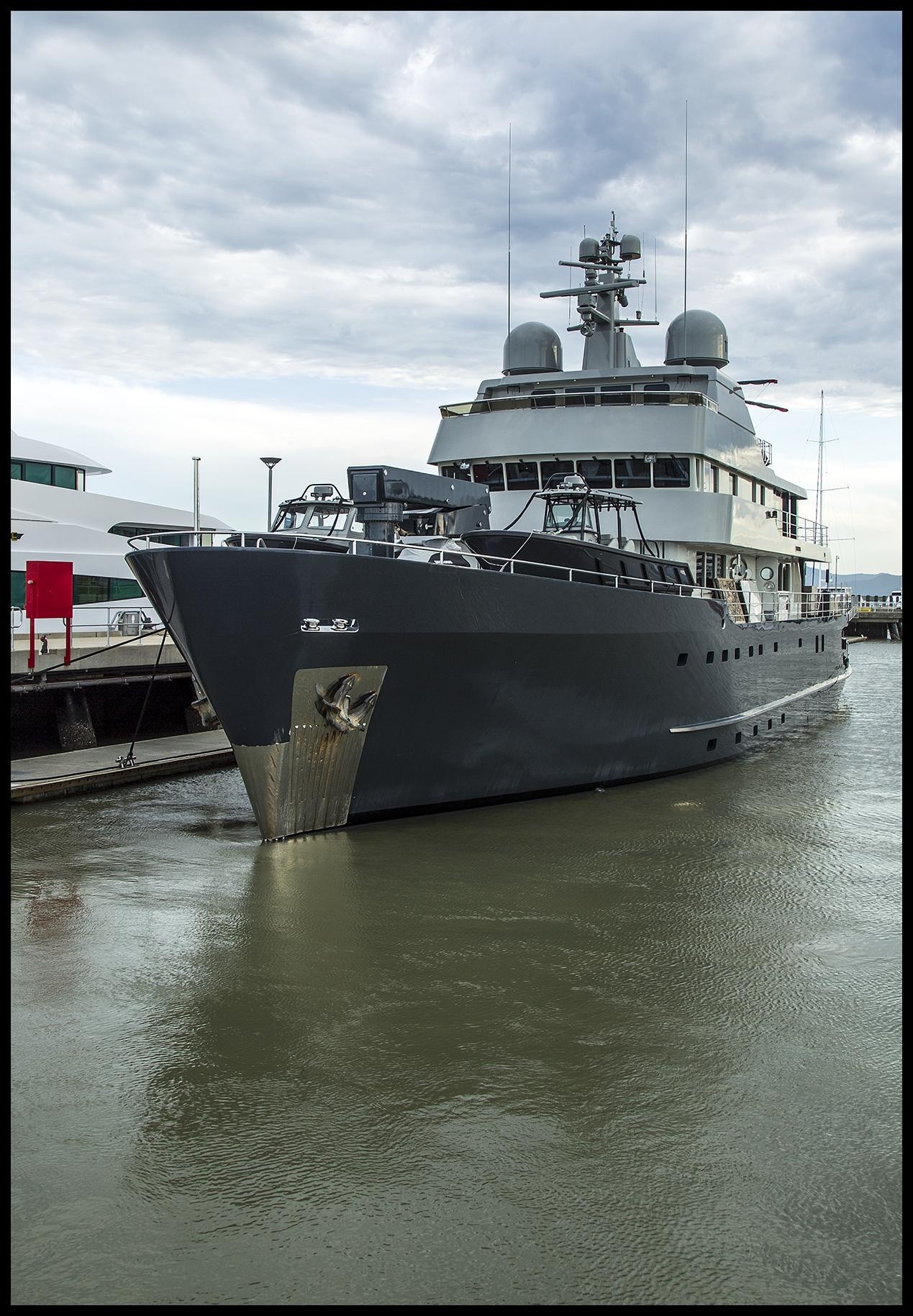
[124,100,855,845]
[9,434,240,758]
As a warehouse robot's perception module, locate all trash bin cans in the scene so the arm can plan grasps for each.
[123,612,139,636]
